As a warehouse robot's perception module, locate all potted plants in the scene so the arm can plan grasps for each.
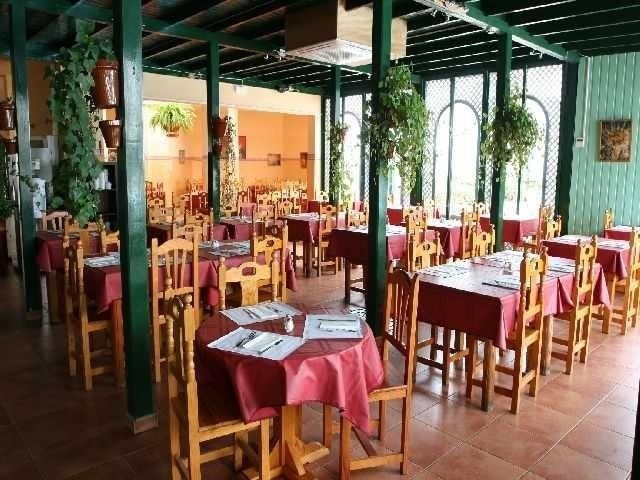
[0,18,545,228]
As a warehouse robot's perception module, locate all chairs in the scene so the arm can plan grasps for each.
[169,256,420,480]
[38,179,499,394]
[386,209,640,414]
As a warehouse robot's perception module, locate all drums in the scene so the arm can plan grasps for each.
[242,307,262,322]
[257,339,284,356]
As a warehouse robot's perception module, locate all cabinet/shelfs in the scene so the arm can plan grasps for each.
[385,214,390,231]
[94,169,113,190]
[439,215,447,223]
[502,258,513,274]
[211,239,220,250]
[283,313,296,334]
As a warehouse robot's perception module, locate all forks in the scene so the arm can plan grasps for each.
[236,329,257,348]
